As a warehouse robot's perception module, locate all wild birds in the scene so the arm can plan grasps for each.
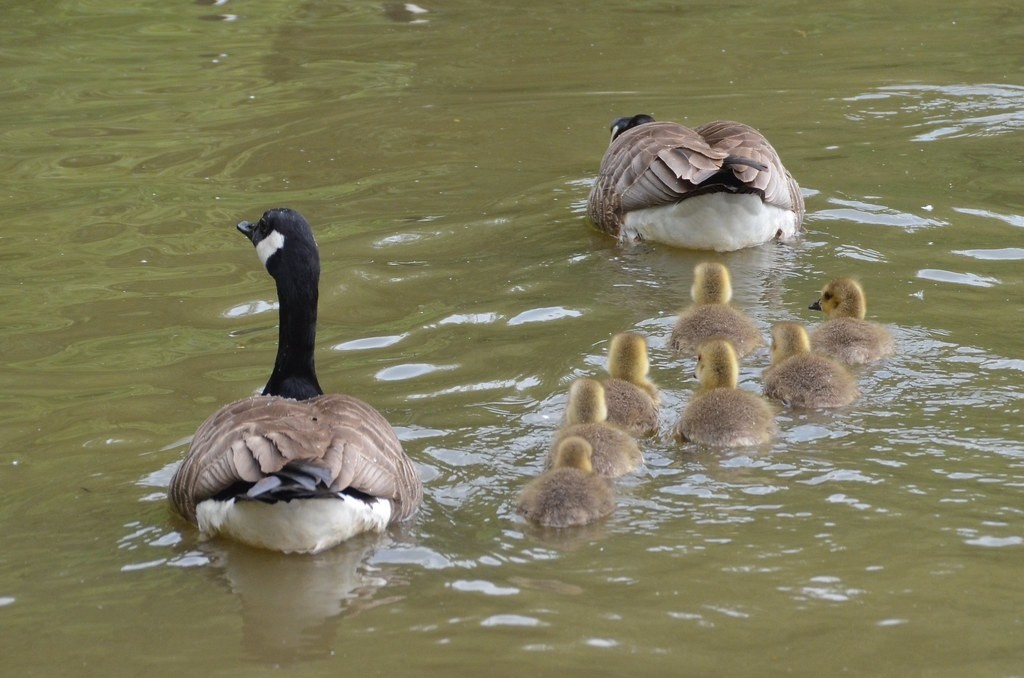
[166,208,422,556]
[515,260,896,529]
[586,114,805,253]
